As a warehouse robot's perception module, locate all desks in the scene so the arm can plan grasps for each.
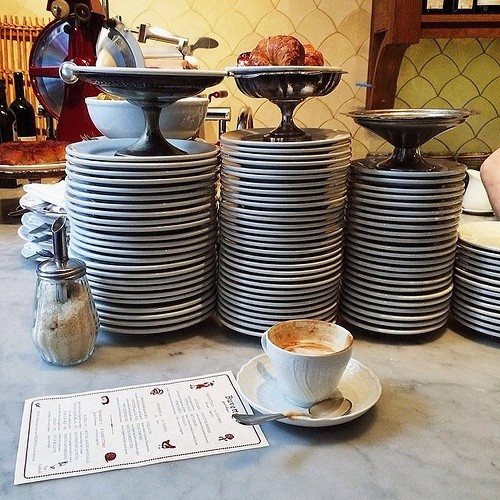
[0,224,500,500]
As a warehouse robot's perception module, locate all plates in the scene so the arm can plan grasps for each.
[236,354,382,427]
[217,127,353,339]
[339,154,467,336]
[66,137,221,333]
[449,169,500,338]
[18,193,69,263]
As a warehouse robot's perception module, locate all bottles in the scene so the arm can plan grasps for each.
[423,0,500,13]
[0,78,18,141]
[10,72,37,142]
[31,217,100,368]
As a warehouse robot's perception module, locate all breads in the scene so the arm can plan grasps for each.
[0,140,73,166]
[237,34,324,67]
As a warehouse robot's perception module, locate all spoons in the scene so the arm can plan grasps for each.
[231,397,353,426]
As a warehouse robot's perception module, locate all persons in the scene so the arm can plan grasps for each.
[480,148,500,222]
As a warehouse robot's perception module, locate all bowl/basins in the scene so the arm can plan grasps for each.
[86,97,207,140]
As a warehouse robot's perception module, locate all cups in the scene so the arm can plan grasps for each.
[261,319,354,407]
